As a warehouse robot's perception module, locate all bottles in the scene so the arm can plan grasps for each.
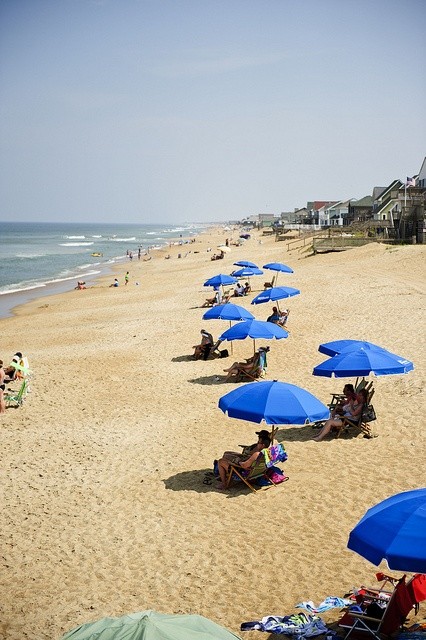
[352,586,358,595]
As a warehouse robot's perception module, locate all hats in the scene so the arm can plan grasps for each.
[255,430,270,437]
[258,436,270,448]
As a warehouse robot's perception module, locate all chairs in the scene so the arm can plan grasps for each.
[245,284,252,295]
[238,427,278,454]
[192,340,223,359]
[327,378,369,414]
[333,387,375,440]
[366,380,374,391]
[263,276,275,292]
[350,572,426,621]
[338,574,412,640]
[224,442,283,494]
[4,359,23,381]
[269,309,290,334]
[207,293,218,306]
[234,351,271,383]
[3,380,28,409]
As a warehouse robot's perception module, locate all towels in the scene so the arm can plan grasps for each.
[240,612,338,640]
[259,442,289,468]
[294,596,353,612]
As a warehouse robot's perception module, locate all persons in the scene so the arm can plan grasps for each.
[75,280,86,290]
[234,282,243,296]
[129,252,132,261]
[110,278,119,286]
[193,330,212,359]
[222,353,259,383]
[125,271,129,284]
[311,389,369,441]
[137,247,141,261]
[225,238,230,247]
[332,385,357,417]
[125,249,129,257]
[268,307,277,322]
[3,356,18,384]
[13,352,22,362]
[216,438,271,488]
[201,293,219,307]
[243,282,251,295]
[0,360,5,413]
[222,430,269,460]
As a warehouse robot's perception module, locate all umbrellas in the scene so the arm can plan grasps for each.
[318,339,392,390]
[203,274,238,303]
[313,347,415,430]
[218,378,331,447]
[60,611,241,640]
[233,261,259,269]
[347,486,426,571]
[231,268,263,290]
[264,264,294,287]
[203,303,255,354]
[239,234,250,243]
[217,245,231,257]
[219,321,288,356]
[252,286,300,315]
